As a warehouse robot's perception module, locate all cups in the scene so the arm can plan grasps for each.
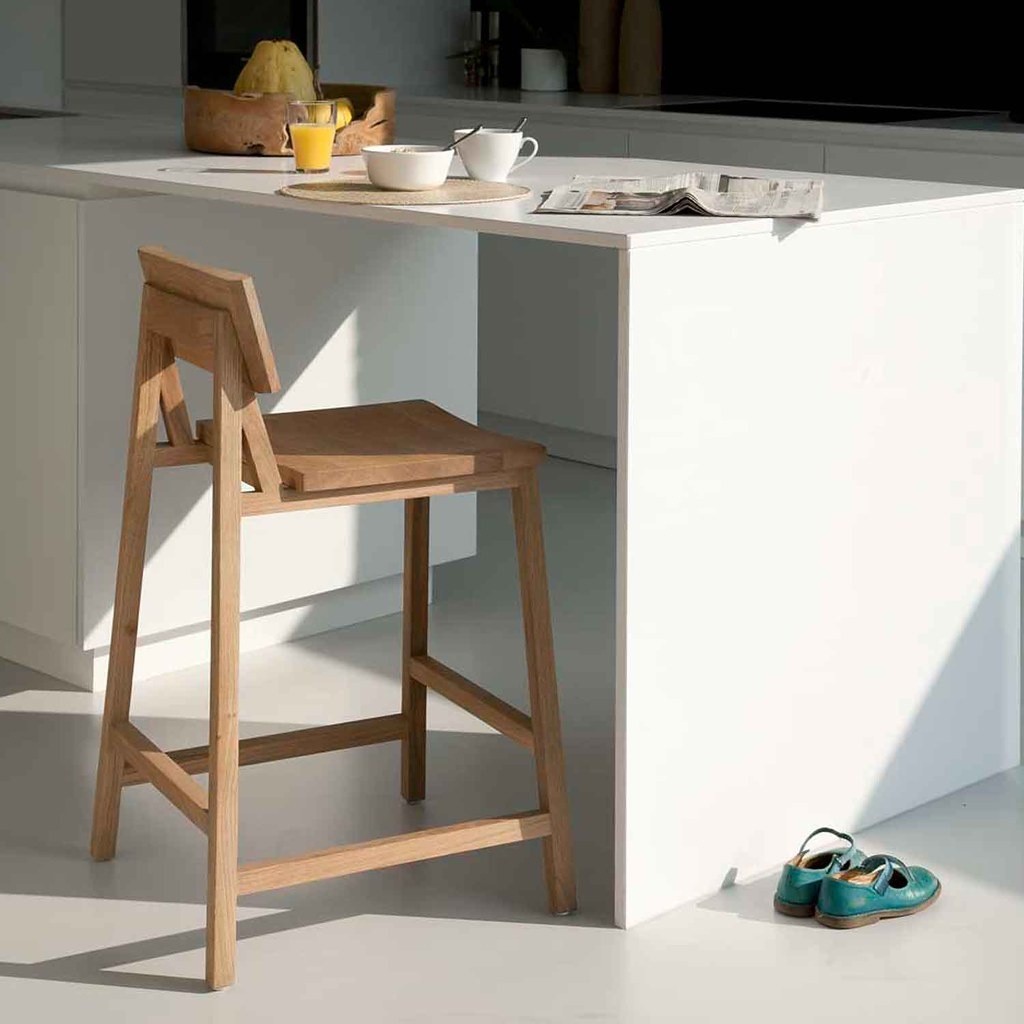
[287,101,336,174]
[454,129,537,180]
[522,48,568,91]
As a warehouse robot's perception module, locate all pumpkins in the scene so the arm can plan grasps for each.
[234,40,355,130]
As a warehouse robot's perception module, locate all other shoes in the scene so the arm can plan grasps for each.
[774,827,867,918]
[814,854,942,930]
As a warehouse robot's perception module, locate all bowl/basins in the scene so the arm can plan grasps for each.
[362,144,453,191]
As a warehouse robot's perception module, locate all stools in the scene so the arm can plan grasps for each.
[90,246,579,990]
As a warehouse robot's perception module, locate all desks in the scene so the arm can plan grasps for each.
[1,109,1024,929]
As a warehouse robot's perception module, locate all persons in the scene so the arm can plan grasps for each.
[582,191,674,211]
